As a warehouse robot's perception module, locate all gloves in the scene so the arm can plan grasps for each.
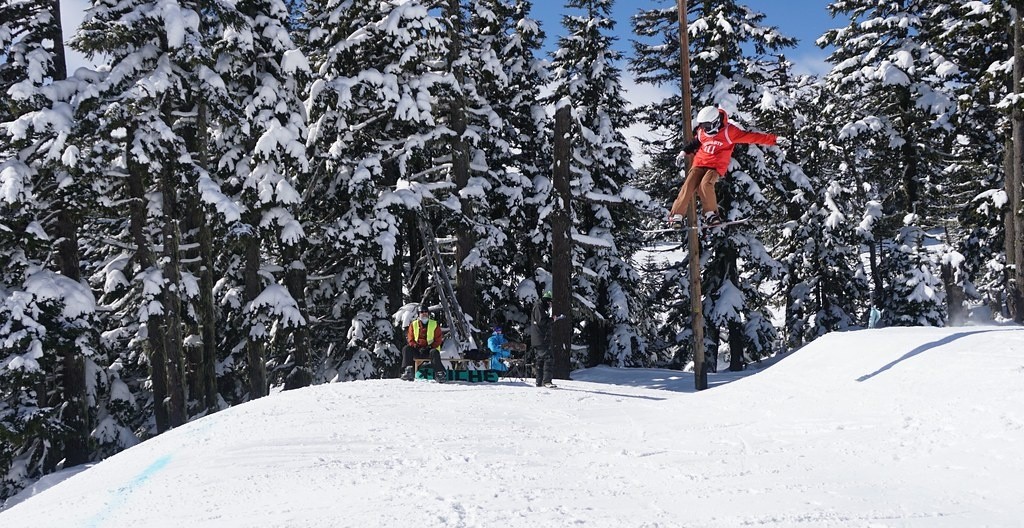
[777,137,791,146]
[676,151,686,165]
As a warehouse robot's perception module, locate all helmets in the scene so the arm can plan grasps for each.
[697,106,721,133]
[542,291,552,298]
[417,305,428,311]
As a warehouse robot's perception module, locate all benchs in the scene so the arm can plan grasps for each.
[414,357,489,379]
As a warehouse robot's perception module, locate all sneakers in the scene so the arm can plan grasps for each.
[671,214,684,229]
[705,211,719,225]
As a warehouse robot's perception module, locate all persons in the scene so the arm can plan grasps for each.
[487,326,511,371]
[530,292,557,388]
[401,304,447,383]
[668,106,791,227]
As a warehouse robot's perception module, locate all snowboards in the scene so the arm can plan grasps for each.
[636,215,754,234]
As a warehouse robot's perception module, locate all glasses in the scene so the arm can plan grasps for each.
[420,311,428,313]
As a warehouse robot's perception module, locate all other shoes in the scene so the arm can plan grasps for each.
[536,382,557,388]
[435,371,447,383]
[401,366,413,380]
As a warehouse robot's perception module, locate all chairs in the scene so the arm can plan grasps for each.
[500,344,526,382]
